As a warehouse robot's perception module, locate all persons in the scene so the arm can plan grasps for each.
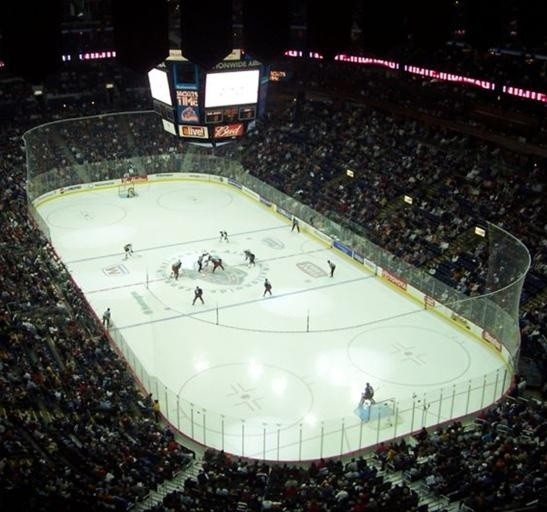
[2,73,547,512]
